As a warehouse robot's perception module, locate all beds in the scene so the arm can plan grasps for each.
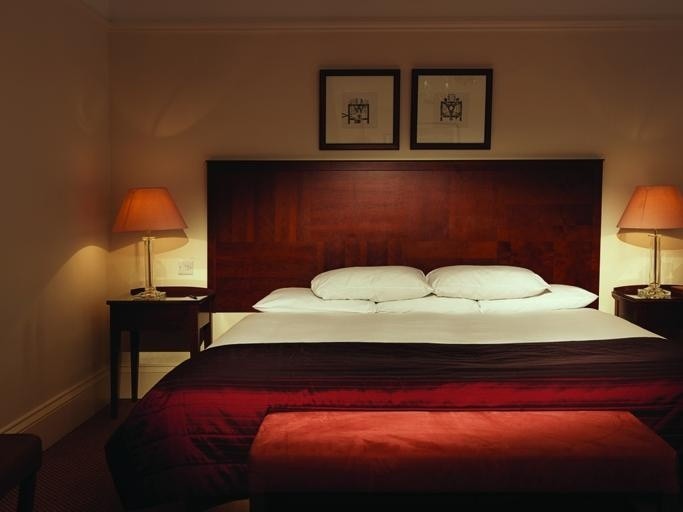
[105,310,683,512]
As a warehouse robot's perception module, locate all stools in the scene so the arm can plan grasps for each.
[0,435,42,512]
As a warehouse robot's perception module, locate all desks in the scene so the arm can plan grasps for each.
[106,287,216,419]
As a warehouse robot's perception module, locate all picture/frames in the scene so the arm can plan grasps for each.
[410,69,493,150]
[319,69,399,150]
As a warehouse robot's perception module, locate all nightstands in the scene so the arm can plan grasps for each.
[611,285,683,338]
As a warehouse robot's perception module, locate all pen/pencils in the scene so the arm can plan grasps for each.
[191,295,198,299]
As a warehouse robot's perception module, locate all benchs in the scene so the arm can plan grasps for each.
[246,411,680,512]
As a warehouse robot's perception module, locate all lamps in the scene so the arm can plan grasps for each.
[112,187,187,299]
[617,185,683,300]
